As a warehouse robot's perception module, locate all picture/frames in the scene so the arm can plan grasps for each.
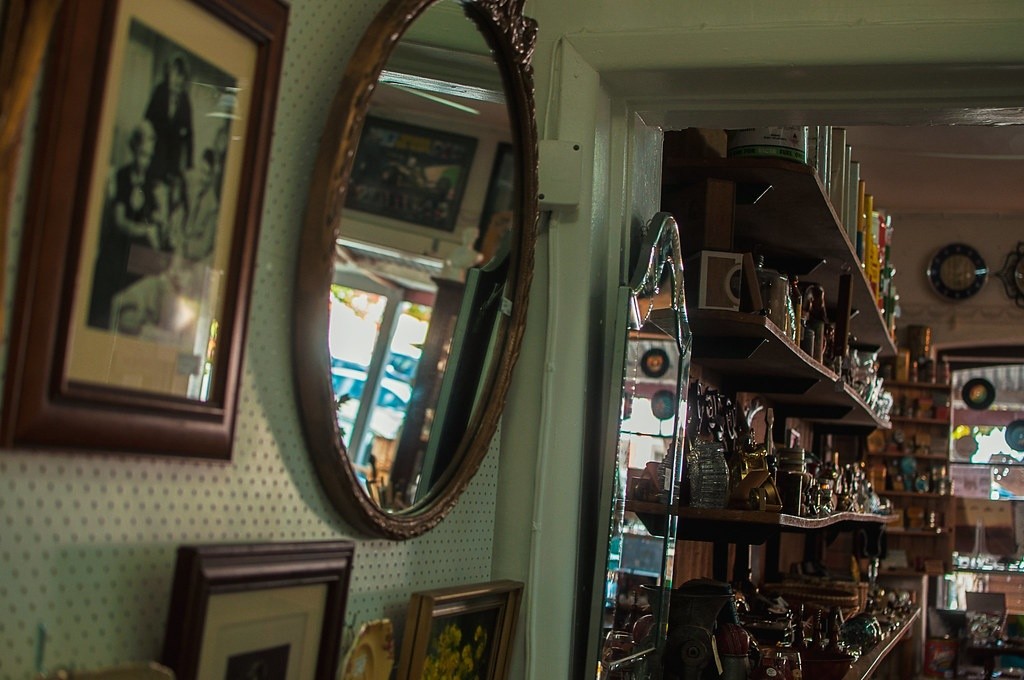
[159,541,357,680]
[395,579,525,680]
[471,139,518,257]
[343,116,480,233]
[1,0,296,460]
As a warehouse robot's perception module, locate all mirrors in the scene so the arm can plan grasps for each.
[296,1,540,540]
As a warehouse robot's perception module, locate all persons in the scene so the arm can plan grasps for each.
[376,155,431,212]
[112,49,217,295]
[421,622,483,680]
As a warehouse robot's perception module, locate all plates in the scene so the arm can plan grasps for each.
[335,618,395,680]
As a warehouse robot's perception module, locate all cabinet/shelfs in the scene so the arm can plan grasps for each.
[648,151,1024,680]
